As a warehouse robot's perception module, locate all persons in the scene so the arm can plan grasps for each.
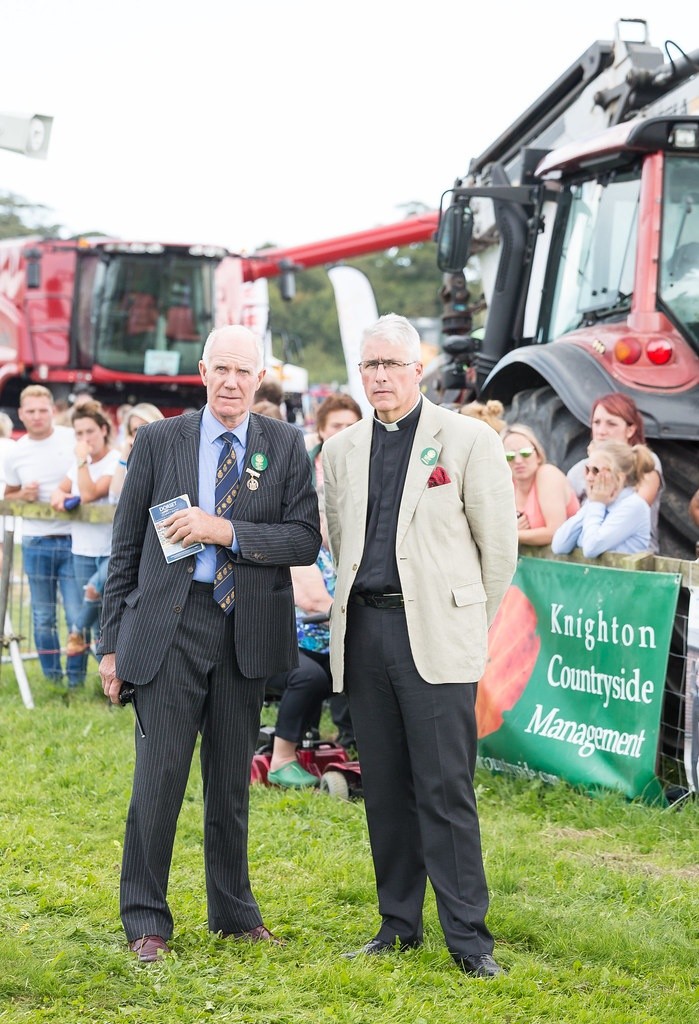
[95,323,317,960]
[320,315,518,980]
[0,384,661,790]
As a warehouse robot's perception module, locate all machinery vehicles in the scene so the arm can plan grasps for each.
[0,225,315,449]
[241,18,699,804]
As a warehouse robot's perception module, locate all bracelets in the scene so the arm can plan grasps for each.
[77,460,87,469]
[118,457,126,466]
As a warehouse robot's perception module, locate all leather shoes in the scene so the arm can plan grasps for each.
[220,925,287,947]
[457,952,507,978]
[131,935,169,961]
[340,935,422,959]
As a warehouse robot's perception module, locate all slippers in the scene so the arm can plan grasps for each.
[267,761,319,788]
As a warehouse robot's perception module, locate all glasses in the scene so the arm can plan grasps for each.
[358,359,416,373]
[506,447,533,461]
[585,464,611,476]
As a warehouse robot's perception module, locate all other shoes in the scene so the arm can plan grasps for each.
[82,584,101,604]
[64,634,85,656]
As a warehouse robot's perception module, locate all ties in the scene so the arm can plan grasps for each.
[213,432,238,617]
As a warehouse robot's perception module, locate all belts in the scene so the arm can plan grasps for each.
[348,593,404,608]
[193,582,213,592]
